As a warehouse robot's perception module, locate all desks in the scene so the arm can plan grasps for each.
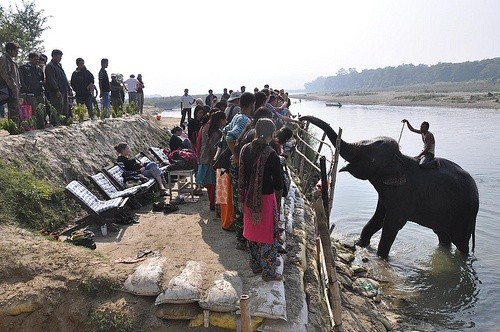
[167,169,201,200]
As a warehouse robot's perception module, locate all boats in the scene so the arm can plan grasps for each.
[325,102,342,107]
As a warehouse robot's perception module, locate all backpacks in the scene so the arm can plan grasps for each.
[66,231,96,251]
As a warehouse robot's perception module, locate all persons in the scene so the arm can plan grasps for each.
[110,74,125,116]
[18,53,47,129]
[180,89,195,125]
[114,142,170,197]
[71,58,94,118]
[99,59,110,118]
[123,75,142,104]
[237,118,283,281]
[188,85,302,253]
[136,74,145,113]
[0,43,21,125]
[170,126,190,151]
[44,49,72,126]
[402,119,440,170]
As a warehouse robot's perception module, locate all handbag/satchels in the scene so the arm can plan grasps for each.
[19,104,33,120]
[281,170,291,197]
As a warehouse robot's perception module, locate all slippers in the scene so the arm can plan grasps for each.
[137,248,152,258]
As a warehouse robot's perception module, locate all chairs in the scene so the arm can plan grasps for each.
[59,146,171,235]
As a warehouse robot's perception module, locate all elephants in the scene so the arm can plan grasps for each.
[298,114,481,263]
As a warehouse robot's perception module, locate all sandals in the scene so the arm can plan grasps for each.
[152,201,179,213]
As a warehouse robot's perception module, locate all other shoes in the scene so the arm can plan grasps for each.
[262,272,283,282]
[279,239,284,245]
[160,188,172,196]
[115,212,134,224]
[253,267,262,274]
[236,242,248,251]
[278,248,288,254]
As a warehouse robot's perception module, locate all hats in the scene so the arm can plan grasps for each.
[111,73,116,77]
[226,90,241,102]
